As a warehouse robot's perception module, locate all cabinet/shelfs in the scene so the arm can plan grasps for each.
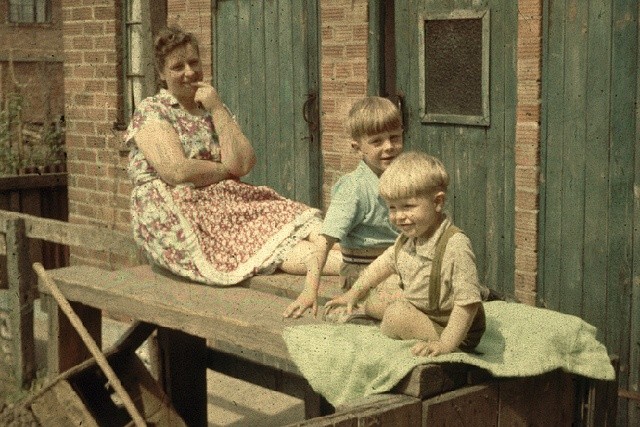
[39,263,621,425]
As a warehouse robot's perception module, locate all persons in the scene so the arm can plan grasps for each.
[322,150,486,356]
[282,94,503,318]
[124,26,342,286]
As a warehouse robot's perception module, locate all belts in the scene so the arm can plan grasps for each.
[342,253,377,265]
